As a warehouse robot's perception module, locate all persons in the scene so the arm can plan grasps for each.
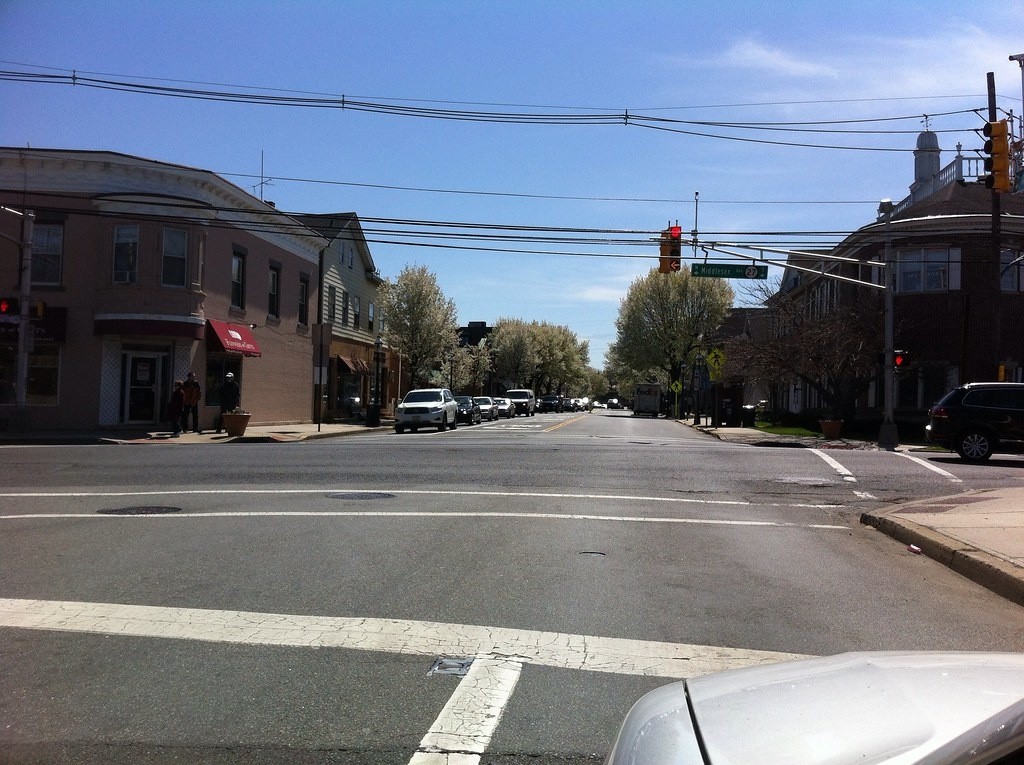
[181,372,202,434]
[214,372,241,433]
[169,380,185,438]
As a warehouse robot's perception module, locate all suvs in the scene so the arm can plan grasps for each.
[394,388,458,434]
[926,381,1024,464]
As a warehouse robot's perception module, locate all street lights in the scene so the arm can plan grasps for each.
[372,335,385,404]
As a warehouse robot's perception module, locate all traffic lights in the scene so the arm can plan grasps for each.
[669,227,681,271]
[984,119,1011,192]
[893,350,909,374]
[0,296,21,316]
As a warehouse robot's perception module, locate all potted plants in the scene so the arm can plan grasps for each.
[222,407,252,437]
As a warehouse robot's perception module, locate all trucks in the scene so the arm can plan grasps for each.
[633,383,661,418]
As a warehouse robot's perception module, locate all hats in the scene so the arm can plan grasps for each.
[226,372,234,378]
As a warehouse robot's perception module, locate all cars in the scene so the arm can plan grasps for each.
[563,396,624,412]
[492,397,516,419]
[453,396,481,425]
[605,651,1023,764]
[537,395,563,413]
[473,396,499,421]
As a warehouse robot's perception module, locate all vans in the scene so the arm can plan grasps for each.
[504,389,535,417]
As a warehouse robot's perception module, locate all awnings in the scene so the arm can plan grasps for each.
[208,318,262,359]
[338,355,374,376]
